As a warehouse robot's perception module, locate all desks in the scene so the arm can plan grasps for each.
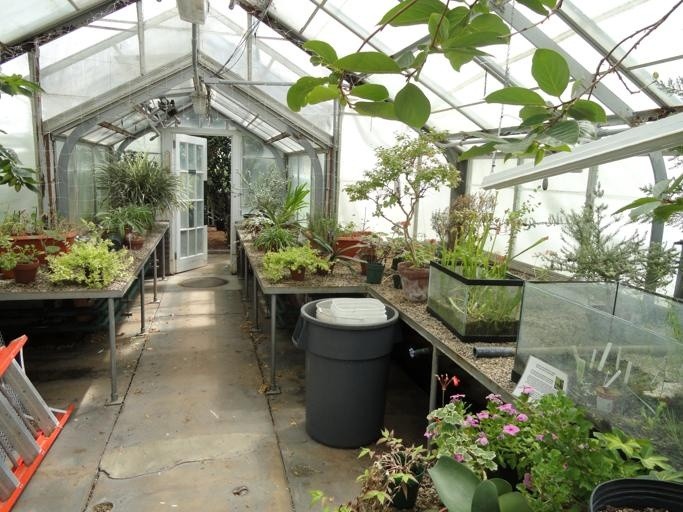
[236,226,683,512]
[0,222,169,406]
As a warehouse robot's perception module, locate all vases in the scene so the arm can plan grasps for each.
[589,478,683,512]
[485,454,520,491]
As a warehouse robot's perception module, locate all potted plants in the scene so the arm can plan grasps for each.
[228,126,463,302]
[355,426,437,512]
[0,147,195,286]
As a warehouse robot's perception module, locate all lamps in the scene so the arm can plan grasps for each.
[176,0,209,115]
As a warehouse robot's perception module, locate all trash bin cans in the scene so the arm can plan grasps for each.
[297,298,400,449]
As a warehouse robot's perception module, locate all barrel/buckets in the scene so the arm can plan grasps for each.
[290,298,399,448]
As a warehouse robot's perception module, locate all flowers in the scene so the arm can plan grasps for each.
[423,383,537,480]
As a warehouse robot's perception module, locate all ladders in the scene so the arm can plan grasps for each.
[1,335,75,512]
